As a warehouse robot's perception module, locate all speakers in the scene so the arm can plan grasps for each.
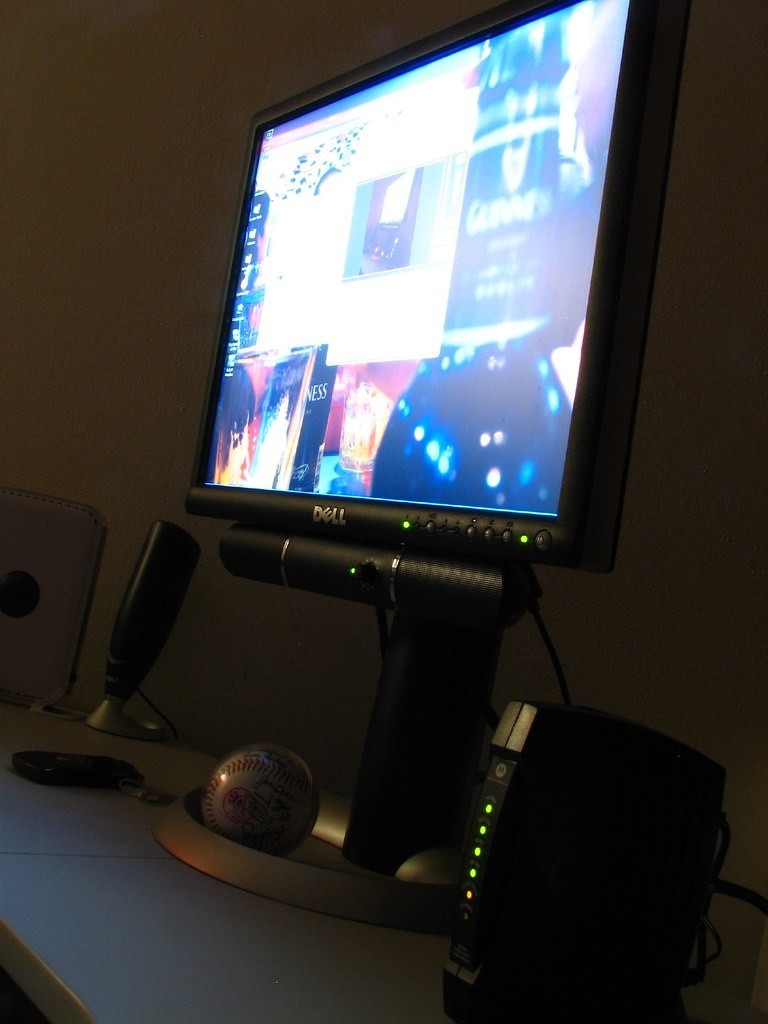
[84,520,202,742]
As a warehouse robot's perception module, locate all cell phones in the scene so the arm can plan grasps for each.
[11,750,143,787]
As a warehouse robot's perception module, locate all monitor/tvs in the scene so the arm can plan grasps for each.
[150,0,694,935]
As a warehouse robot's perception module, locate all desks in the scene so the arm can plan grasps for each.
[0,704,768,1024]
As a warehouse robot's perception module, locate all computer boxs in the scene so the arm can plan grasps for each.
[443,697,728,1024]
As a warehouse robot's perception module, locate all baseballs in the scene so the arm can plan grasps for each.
[201,741,323,860]
